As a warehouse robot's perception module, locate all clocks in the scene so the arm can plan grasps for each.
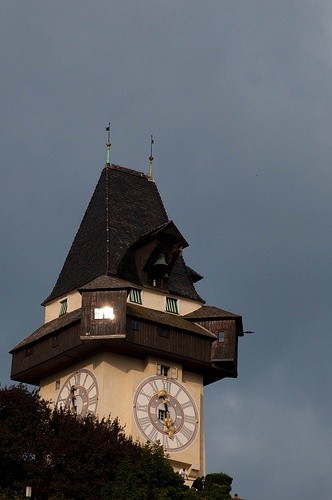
[133,375,200,453]
[53,367,100,422]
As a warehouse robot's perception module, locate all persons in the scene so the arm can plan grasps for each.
[192,476,205,491]
[178,467,189,480]
